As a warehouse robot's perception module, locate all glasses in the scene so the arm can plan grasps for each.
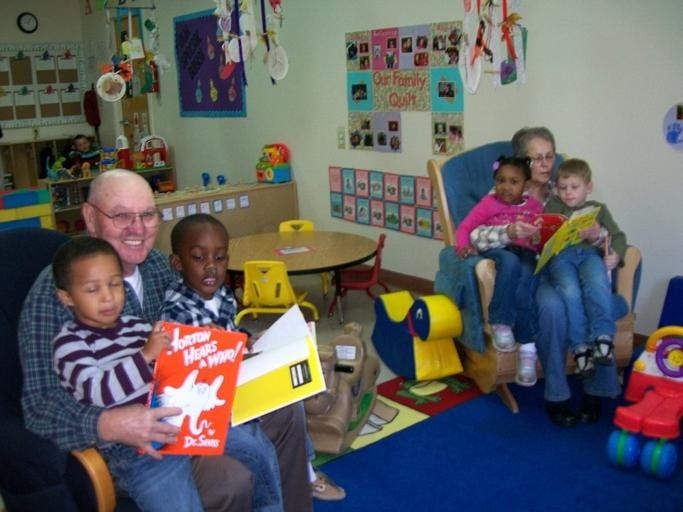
[89,203,164,228]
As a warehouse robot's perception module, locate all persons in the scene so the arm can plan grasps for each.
[470,127,622,426]
[456,155,544,387]
[158,214,346,501]
[542,159,626,373]
[73,134,101,167]
[18,168,312,511]
[52,237,284,511]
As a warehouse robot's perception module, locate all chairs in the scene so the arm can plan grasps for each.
[422,138,645,415]
[322,232,393,319]
[232,255,320,332]
[0,225,146,512]
[276,219,334,298]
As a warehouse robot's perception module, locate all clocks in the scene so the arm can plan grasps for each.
[15,11,39,35]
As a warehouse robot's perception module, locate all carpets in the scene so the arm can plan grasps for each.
[262,342,683,511]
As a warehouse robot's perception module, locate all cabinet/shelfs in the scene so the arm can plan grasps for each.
[0,136,96,190]
[35,165,179,240]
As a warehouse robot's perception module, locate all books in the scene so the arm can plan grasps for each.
[137,322,248,458]
[534,205,601,274]
[230,305,326,428]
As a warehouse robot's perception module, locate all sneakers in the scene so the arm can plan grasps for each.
[579,394,602,424]
[548,400,578,426]
[573,338,613,378]
[311,473,346,501]
[515,349,538,387]
[489,324,516,353]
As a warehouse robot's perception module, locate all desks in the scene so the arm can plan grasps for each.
[217,228,379,326]
